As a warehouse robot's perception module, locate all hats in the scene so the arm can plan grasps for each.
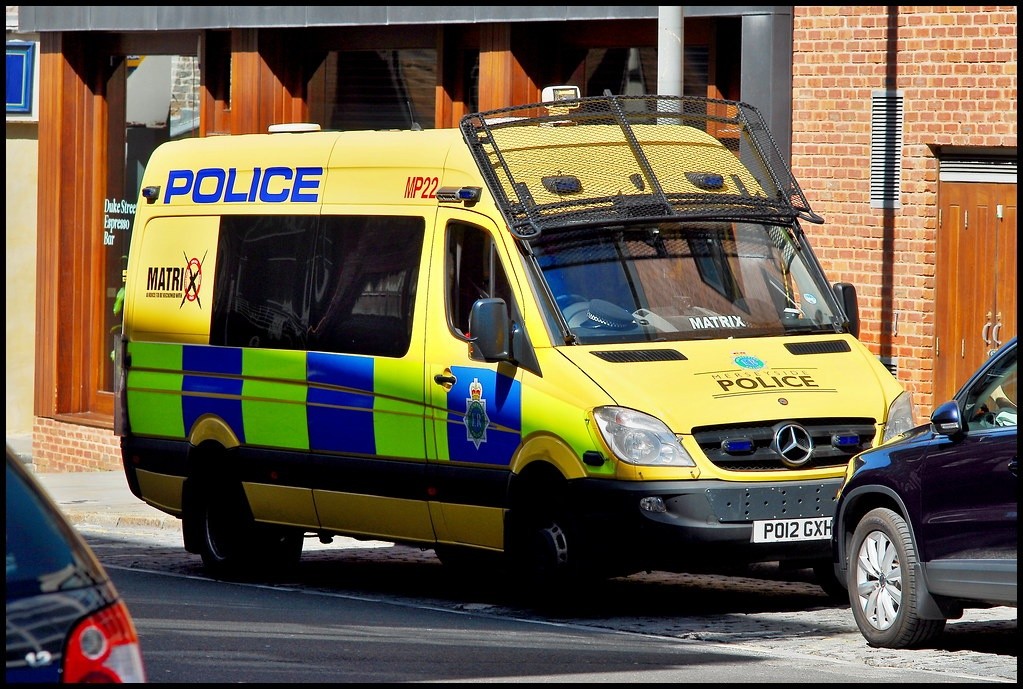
[580,299,634,329]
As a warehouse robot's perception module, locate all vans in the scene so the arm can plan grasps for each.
[110,88,913,625]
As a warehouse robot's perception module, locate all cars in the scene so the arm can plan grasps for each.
[2,453,146,686]
[833,335,1018,651]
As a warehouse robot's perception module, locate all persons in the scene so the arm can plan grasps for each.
[485,251,521,326]
